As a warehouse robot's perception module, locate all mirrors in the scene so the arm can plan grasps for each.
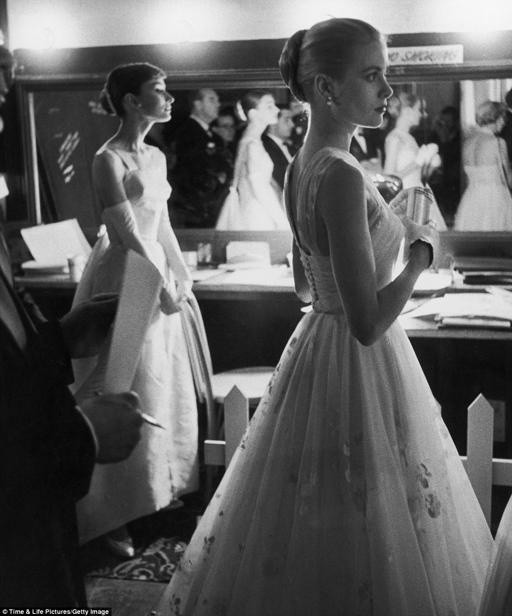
[15,62,512,264]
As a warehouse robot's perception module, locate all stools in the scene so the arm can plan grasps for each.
[209,364,277,477]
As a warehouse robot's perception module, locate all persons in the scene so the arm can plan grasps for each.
[156,17,494,616]
[175,86,512,231]
[0,46,143,616]
[65,62,215,557]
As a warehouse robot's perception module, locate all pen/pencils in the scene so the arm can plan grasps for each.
[91,386,169,433]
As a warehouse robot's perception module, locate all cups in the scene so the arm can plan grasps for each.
[68,257,83,283]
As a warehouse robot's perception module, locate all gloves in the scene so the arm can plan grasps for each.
[105,199,183,316]
[158,205,193,302]
[387,185,440,271]
[414,142,442,169]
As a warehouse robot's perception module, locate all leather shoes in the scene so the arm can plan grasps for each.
[107,533,135,558]
